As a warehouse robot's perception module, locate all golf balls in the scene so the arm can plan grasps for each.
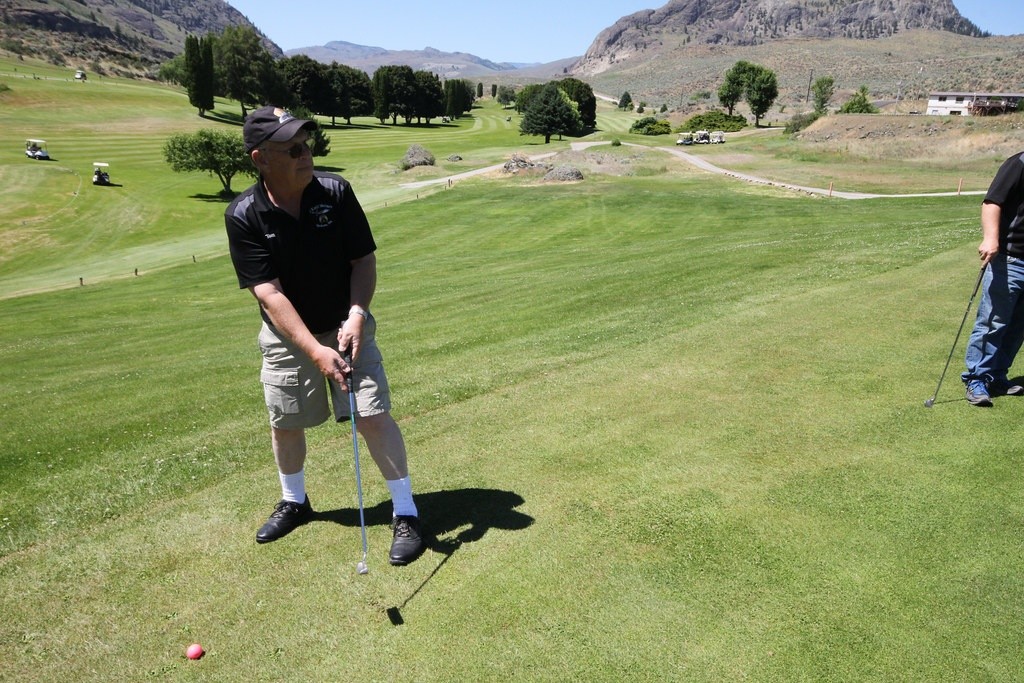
[187,643,202,660]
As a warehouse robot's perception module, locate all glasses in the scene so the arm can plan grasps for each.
[252,137,315,159]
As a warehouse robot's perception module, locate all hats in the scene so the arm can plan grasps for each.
[243,105,317,154]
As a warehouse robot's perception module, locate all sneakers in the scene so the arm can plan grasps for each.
[256,493,313,543]
[965,374,993,407]
[988,376,1023,395]
[389,515,423,565]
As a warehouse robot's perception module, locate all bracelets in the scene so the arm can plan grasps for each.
[348,308,368,321]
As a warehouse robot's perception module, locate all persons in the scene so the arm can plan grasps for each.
[960,152,1024,406]
[224,107,428,566]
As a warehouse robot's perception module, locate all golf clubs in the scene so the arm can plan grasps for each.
[925,263,987,407]
[342,351,368,574]
